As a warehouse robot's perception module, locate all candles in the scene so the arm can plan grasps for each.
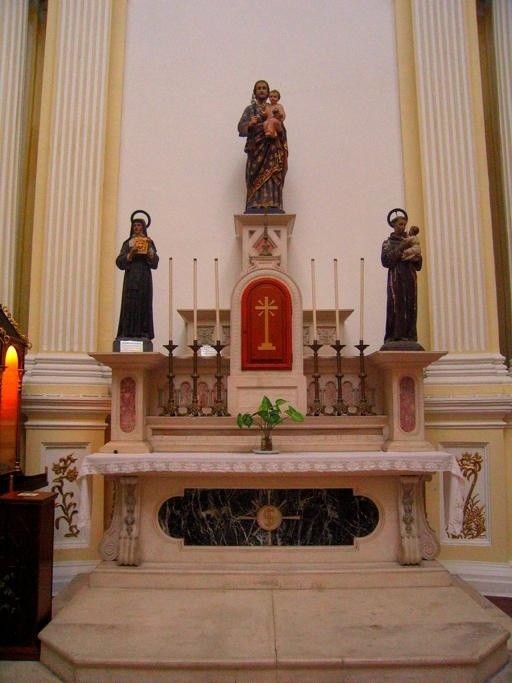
[164,255,223,345]
[308,255,367,342]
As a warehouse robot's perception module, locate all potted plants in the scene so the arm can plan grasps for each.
[234,395,307,449]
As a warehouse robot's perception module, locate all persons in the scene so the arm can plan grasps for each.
[257,87,286,139]
[399,224,421,261]
[235,78,289,210]
[380,206,422,341]
[112,218,161,341]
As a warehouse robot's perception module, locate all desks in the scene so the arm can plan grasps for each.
[1,489,61,665]
[80,451,457,570]
